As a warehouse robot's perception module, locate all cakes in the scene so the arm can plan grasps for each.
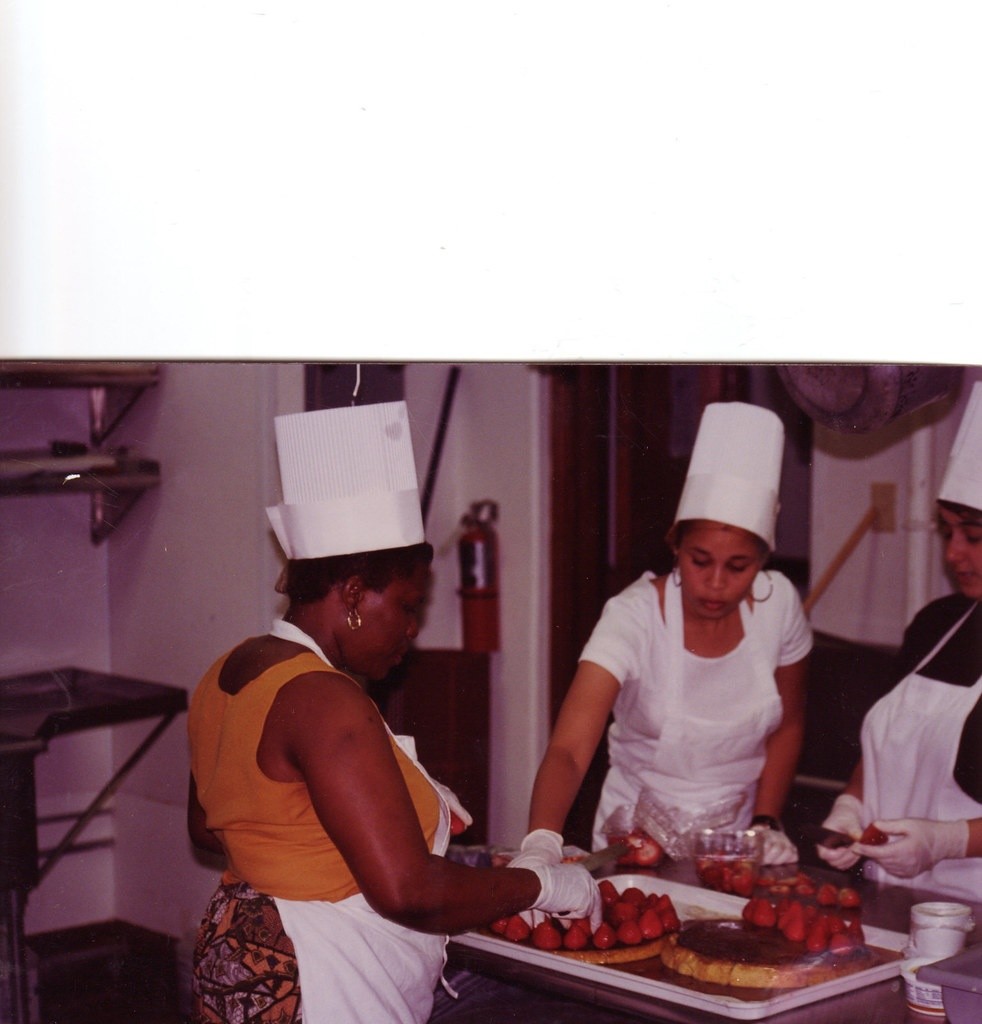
[486,878,681,964]
[659,893,874,990]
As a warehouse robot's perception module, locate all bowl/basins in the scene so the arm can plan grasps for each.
[899,960,946,1015]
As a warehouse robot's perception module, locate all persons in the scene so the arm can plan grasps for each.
[816,498,982,896]
[507,519,812,873]
[186,542,600,1024]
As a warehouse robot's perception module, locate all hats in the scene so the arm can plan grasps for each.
[675,402,784,552]
[264,401,425,559]
[936,380,982,511]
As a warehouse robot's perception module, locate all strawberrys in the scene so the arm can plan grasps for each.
[601,823,663,866]
[859,823,887,845]
[699,846,861,907]
[448,811,465,835]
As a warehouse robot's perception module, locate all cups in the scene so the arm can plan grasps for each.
[912,902,971,958]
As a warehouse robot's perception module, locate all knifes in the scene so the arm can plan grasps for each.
[578,845,628,870]
[794,822,851,848]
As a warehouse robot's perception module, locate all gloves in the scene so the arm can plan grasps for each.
[815,795,865,870]
[851,817,968,879]
[748,825,799,865]
[505,829,565,929]
[434,782,475,826]
[528,863,602,934]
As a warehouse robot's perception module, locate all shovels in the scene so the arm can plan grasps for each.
[581,842,627,871]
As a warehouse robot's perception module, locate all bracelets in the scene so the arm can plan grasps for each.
[752,815,780,832]
